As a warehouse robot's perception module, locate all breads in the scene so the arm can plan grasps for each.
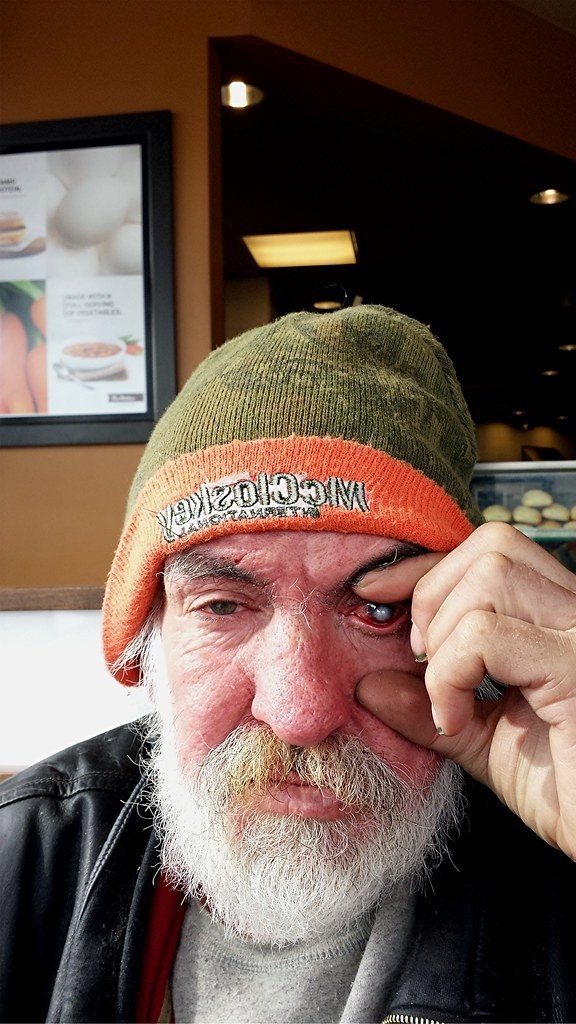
[483,490,576,528]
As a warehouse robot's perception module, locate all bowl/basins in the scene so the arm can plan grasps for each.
[55,335,127,371]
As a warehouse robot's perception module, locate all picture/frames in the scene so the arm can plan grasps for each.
[0,104,177,449]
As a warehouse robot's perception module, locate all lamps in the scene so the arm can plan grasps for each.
[243,211,359,268]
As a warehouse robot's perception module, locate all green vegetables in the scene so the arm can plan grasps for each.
[1,281,42,342]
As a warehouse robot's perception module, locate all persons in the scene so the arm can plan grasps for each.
[1,305,576,1023]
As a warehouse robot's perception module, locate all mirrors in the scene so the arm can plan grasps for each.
[204,33,576,464]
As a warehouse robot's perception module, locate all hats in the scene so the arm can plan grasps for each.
[101,304,486,687]
